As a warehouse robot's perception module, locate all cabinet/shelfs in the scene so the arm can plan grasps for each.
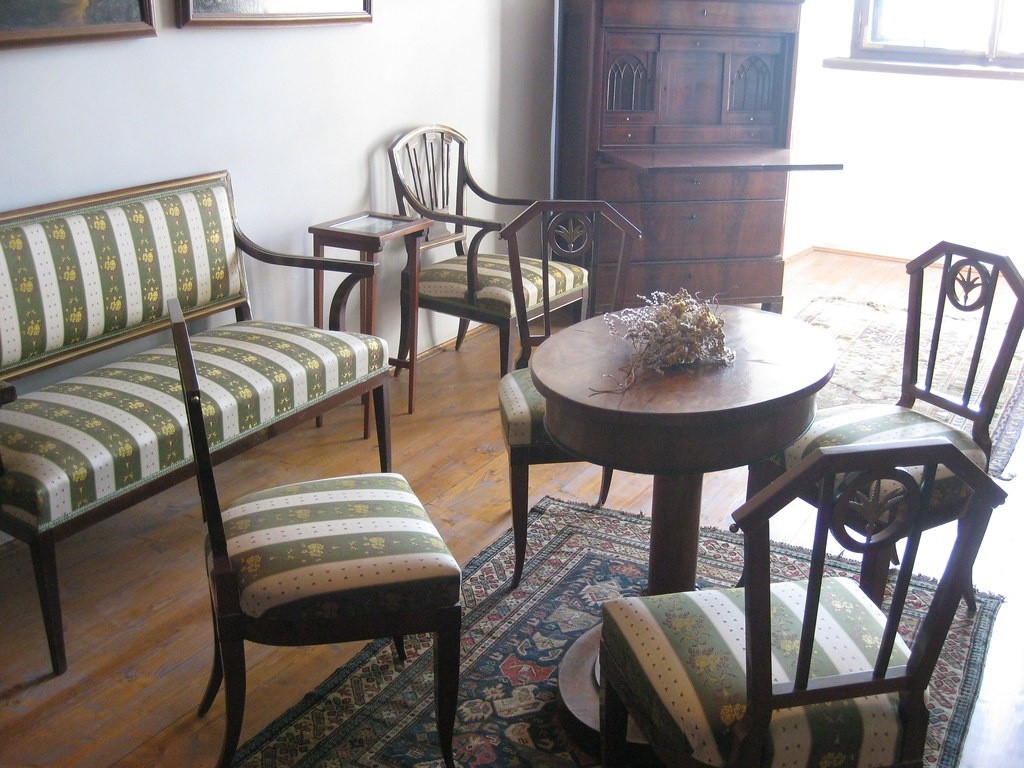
[555,0,844,314]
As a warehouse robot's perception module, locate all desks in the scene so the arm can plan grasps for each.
[527,302,837,768]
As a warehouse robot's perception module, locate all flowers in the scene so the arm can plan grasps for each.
[589,286,737,394]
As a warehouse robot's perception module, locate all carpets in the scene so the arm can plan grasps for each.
[218,494,1006,768]
[710,245,1024,482]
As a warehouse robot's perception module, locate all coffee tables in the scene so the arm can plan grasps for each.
[308,210,434,440]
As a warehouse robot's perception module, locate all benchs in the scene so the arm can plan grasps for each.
[0,169,392,675]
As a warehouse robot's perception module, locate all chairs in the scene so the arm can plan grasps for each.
[498,200,642,589]
[166,297,466,768]
[733,242,1024,612]
[597,435,1008,768]
[387,123,590,380]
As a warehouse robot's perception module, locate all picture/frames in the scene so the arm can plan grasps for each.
[175,0,373,29]
[0,0,158,52]
